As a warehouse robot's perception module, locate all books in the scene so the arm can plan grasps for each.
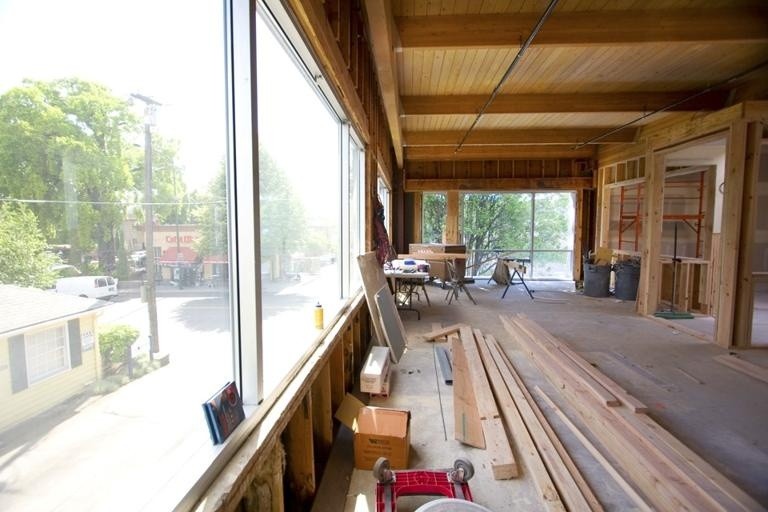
[201,382,245,445]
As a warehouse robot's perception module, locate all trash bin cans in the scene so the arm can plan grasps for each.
[583,259,641,300]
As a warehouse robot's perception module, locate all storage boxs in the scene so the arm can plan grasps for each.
[331,390,412,474]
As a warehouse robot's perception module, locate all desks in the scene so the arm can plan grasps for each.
[383,269,430,320]
[488,256,534,298]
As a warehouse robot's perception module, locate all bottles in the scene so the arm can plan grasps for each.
[315,301,324,330]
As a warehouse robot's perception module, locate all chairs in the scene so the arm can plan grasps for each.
[443,255,477,306]
